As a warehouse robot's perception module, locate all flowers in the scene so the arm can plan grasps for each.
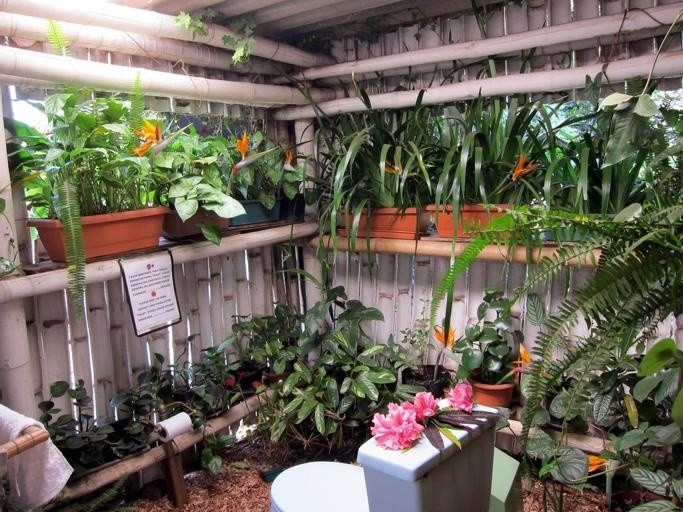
[131,113,310,246]
[369,382,504,459]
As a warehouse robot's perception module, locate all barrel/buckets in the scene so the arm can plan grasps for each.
[268,405,498,512]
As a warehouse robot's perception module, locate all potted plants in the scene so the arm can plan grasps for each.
[308,73,442,241]
[445,299,525,412]
[2,70,168,325]
[541,78,653,241]
[431,87,529,237]
